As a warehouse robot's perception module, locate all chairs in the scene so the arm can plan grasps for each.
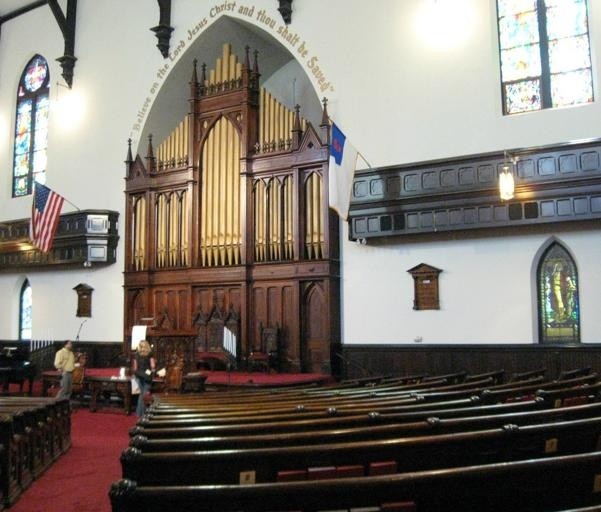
[248,322,280,374]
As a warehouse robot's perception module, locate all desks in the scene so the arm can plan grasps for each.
[145,328,199,376]
[41,372,87,407]
[88,375,131,414]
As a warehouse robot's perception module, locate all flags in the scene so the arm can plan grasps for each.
[327,120,360,223]
[28,179,64,254]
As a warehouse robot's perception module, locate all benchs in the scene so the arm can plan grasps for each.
[189,300,241,370]
[0,396,72,512]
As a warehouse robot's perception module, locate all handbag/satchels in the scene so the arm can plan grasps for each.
[129,374,140,395]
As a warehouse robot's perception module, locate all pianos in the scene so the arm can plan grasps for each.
[0,339,36,396]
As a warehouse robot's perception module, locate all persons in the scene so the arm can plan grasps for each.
[54,340,75,413]
[130,340,156,418]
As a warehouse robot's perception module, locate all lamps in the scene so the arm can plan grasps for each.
[498,149,517,203]
